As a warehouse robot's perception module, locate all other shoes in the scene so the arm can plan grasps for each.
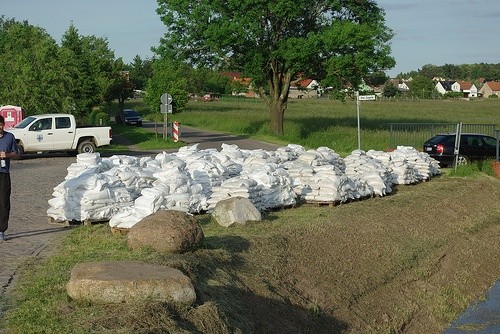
[0,232,5,240]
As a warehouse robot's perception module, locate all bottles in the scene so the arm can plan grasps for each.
[1,150,6,168]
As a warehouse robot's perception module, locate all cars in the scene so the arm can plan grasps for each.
[423,133,500,168]
[115,109,142,128]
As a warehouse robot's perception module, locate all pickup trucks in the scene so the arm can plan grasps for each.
[4,114,113,159]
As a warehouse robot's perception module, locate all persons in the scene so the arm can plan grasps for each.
[0,114,18,241]
